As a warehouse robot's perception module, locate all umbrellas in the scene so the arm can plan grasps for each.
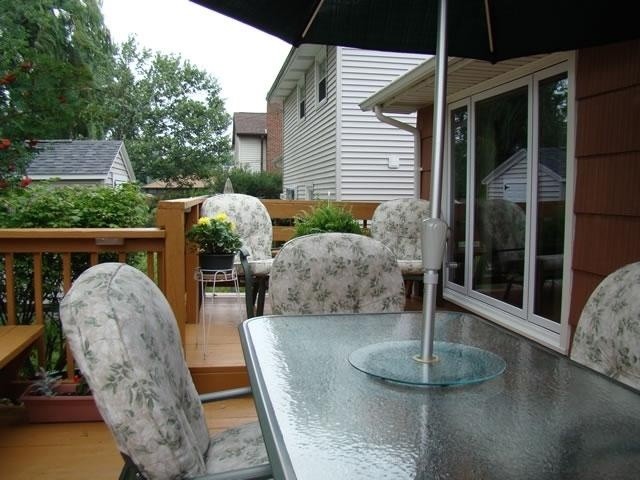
[185,0,639,361]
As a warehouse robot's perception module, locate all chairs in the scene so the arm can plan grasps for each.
[198,193,274,318]
[369,199,444,301]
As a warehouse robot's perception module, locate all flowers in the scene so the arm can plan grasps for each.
[186,213,242,254]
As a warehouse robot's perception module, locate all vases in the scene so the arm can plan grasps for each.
[197,251,237,275]
